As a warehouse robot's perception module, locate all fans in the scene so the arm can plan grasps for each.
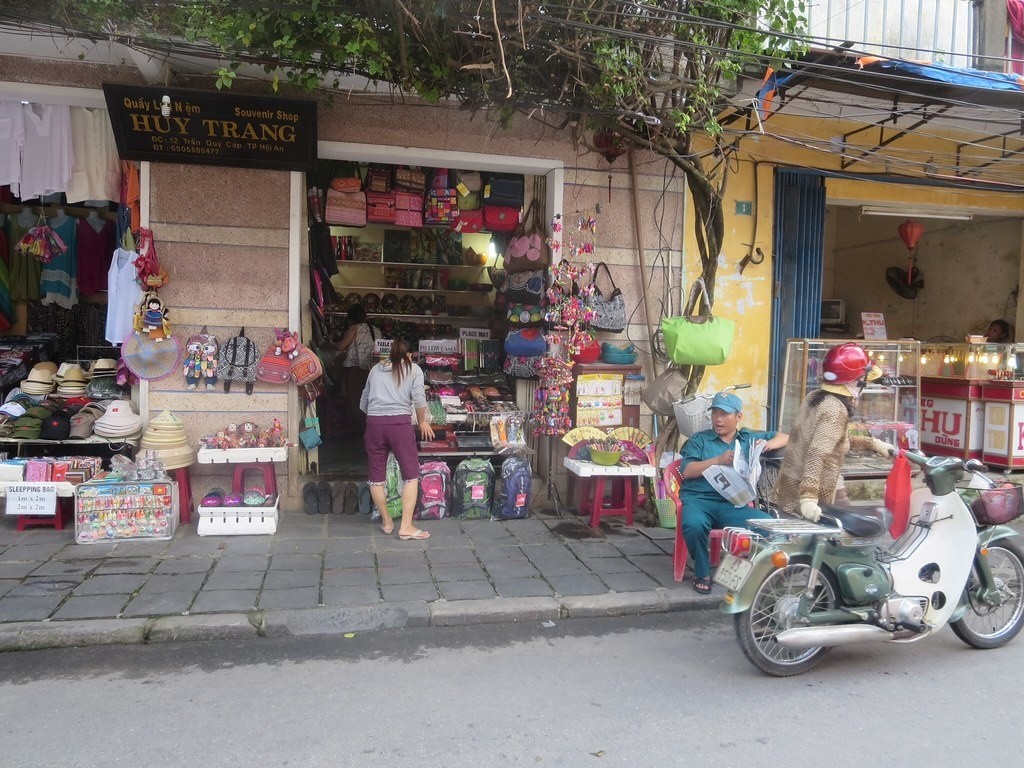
[885,266,925,300]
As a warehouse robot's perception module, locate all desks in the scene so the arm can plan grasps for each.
[0,436,139,457]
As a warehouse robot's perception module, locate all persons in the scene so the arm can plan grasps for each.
[776,342,895,522]
[333,304,375,440]
[984,320,1009,344]
[0,206,115,311]
[360,339,435,541]
[679,392,789,594]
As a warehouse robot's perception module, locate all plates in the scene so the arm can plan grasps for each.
[333,293,432,311]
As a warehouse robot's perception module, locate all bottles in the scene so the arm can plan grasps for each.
[332,236,353,261]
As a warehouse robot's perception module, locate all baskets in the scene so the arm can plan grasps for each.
[955,480,1024,526]
[672,394,713,439]
[650,495,676,528]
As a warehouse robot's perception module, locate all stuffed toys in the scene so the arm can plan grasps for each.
[200,418,298,449]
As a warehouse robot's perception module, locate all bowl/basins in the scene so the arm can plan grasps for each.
[564,344,600,364]
[601,354,636,364]
[469,283,493,292]
[588,444,621,465]
[448,279,467,290]
[468,306,489,316]
[602,342,634,354]
[446,305,468,315]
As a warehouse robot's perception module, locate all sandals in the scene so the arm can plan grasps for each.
[692,574,712,594]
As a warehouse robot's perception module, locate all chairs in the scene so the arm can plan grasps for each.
[663,458,754,581]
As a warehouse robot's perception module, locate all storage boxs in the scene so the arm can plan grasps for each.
[197,438,289,465]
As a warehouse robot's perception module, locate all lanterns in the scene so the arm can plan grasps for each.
[899,218,923,285]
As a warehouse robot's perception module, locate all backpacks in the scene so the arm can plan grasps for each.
[372,452,533,520]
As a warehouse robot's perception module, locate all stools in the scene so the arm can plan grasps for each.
[16,496,72,531]
[231,461,280,510]
[174,466,195,525]
[578,476,634,528]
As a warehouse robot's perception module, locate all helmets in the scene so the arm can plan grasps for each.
[822,342,874,384]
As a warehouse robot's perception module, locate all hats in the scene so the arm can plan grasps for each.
[121,329,181,381]
[707,392,742,413]
[0,359,142,440]
[136,409,197,472]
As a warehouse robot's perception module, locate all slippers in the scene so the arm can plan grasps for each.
[381,524,395,535]
[398,530,430,541]
[303,480,372,515]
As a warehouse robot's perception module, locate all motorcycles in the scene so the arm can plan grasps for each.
[713,447,1022,677]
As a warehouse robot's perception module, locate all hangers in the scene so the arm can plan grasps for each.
[117,238,131,260]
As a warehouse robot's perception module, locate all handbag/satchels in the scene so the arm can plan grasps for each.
[884,449,911,539]
[183,325,322,402]
[640,369,688,415]
[584,263,626,334]
[660,278,737,365]
[486,252,507,289]
[420,363,510,394]
[305,223,340,401]
[298,398,323,452]
[501,200,549,380]
[325,162,524,234]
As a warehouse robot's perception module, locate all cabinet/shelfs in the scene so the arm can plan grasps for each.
[327,259,493,320]
[777,337,922,479]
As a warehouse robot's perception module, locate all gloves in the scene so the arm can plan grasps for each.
[799,499,823,523]
[875,439,895,458]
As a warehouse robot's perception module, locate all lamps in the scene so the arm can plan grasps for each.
[857,206,976,222]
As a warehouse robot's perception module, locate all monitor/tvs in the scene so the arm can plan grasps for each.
[820,299,845,325]
